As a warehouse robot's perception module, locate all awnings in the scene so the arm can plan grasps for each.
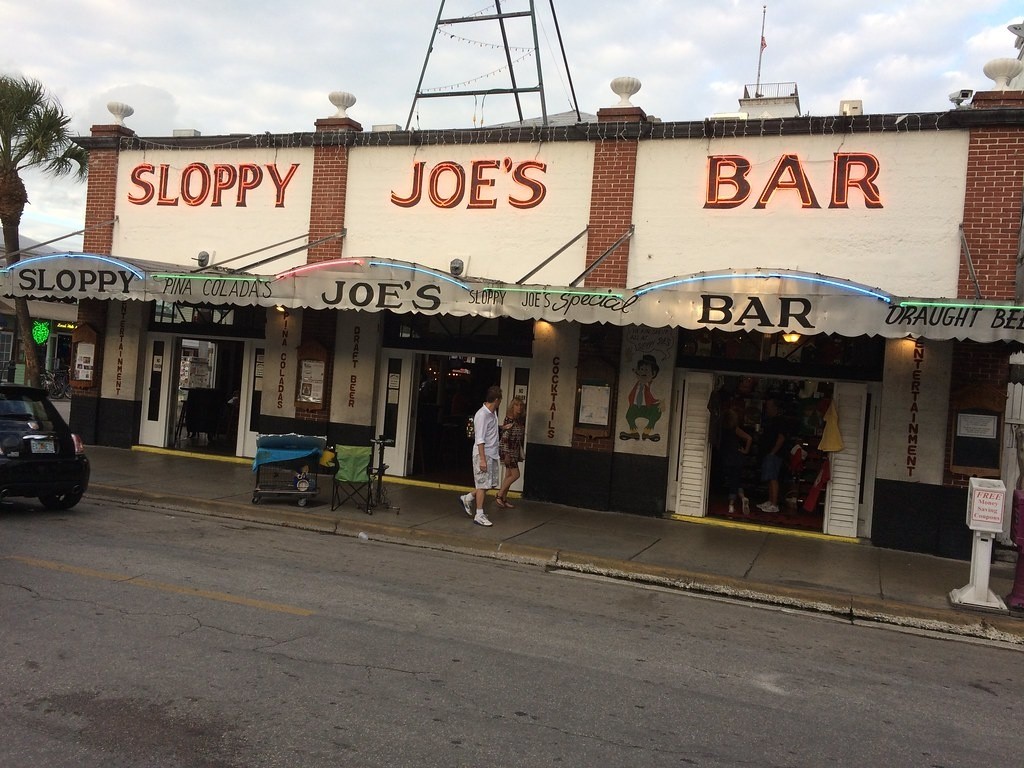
[0,217,1024,344]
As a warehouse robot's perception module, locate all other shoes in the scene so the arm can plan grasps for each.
[742,497,750,514]
[756,501,771,509]
[762,505,779,513]
[729,504,735,512]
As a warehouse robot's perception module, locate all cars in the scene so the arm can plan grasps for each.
[0,380,92,510]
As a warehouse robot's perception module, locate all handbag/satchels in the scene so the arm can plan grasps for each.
[518,446,526,462]
[466,417,503,440]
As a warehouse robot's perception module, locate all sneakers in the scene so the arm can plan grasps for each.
[457,494,472,516]
[473,514,493,526]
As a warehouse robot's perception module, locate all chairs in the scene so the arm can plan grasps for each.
[329,443,376,517]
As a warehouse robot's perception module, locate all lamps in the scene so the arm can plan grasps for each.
[782,332,801,343]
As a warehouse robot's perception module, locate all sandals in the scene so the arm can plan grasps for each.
[504,500,514,509]
[494,494,505,507]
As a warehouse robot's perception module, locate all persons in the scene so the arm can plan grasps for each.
[719,399,785,515]
[492,397,527,508]
[458,386,514,526]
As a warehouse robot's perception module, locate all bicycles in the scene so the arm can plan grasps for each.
[40,366,72,400]
